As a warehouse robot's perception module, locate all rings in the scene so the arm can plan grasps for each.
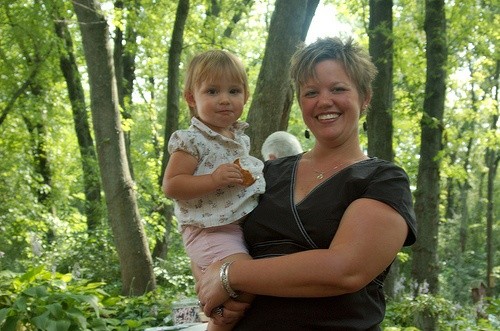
[215,305,223,317]
[198,300,203,307]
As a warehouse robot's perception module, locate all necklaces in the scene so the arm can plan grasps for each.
[303,152,367,179]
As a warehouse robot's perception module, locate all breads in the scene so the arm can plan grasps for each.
[233,158,257,186]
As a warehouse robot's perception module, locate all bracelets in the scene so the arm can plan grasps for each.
[219,261,245,300]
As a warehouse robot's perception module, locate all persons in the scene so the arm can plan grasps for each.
[195,36,419,331]
[162,49,266,331]
[260,131,304,162]
